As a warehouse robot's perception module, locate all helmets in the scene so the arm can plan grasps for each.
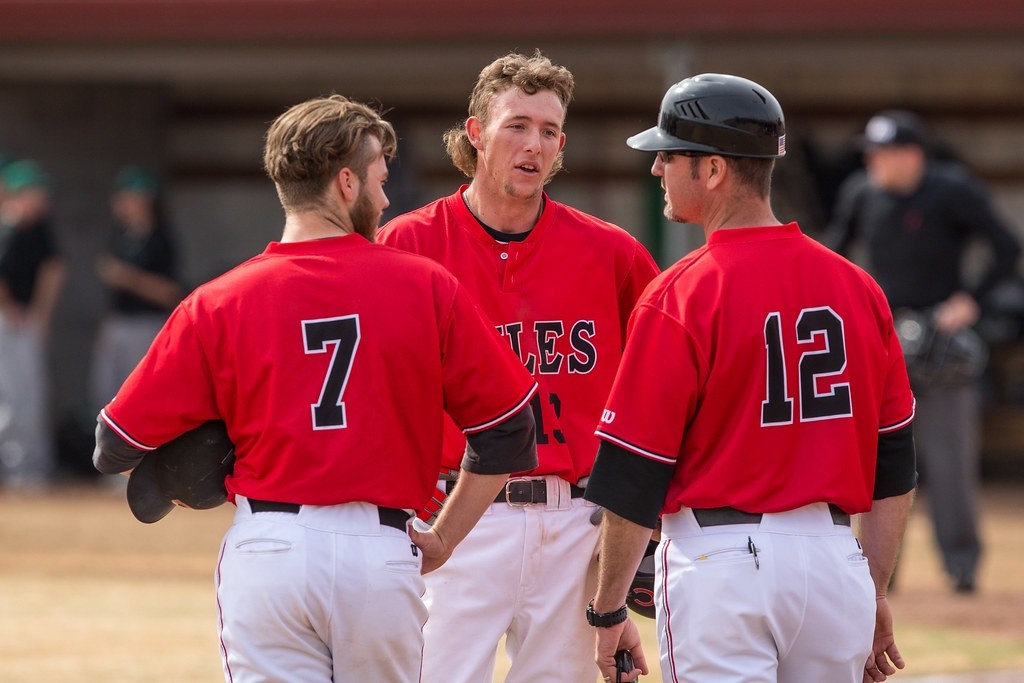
[625,72,787,158]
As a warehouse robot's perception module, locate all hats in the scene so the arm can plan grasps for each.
[1,160,47,191]
[854,111,926,142]
[117,169,154,193]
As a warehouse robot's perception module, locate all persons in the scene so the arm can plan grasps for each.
[377,52,666,683]
[582,74,919,683]
[835,109,999,596]
[96,161,182,493]
[90,95,541,683]
[0,161,64,500]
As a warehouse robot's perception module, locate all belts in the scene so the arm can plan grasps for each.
[692,503,850,527]
[249,497,412,532]
[446,480,586,509]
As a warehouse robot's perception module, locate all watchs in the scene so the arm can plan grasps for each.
[586,598,628,627]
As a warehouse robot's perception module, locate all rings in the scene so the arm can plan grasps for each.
[602,676,610,681]
